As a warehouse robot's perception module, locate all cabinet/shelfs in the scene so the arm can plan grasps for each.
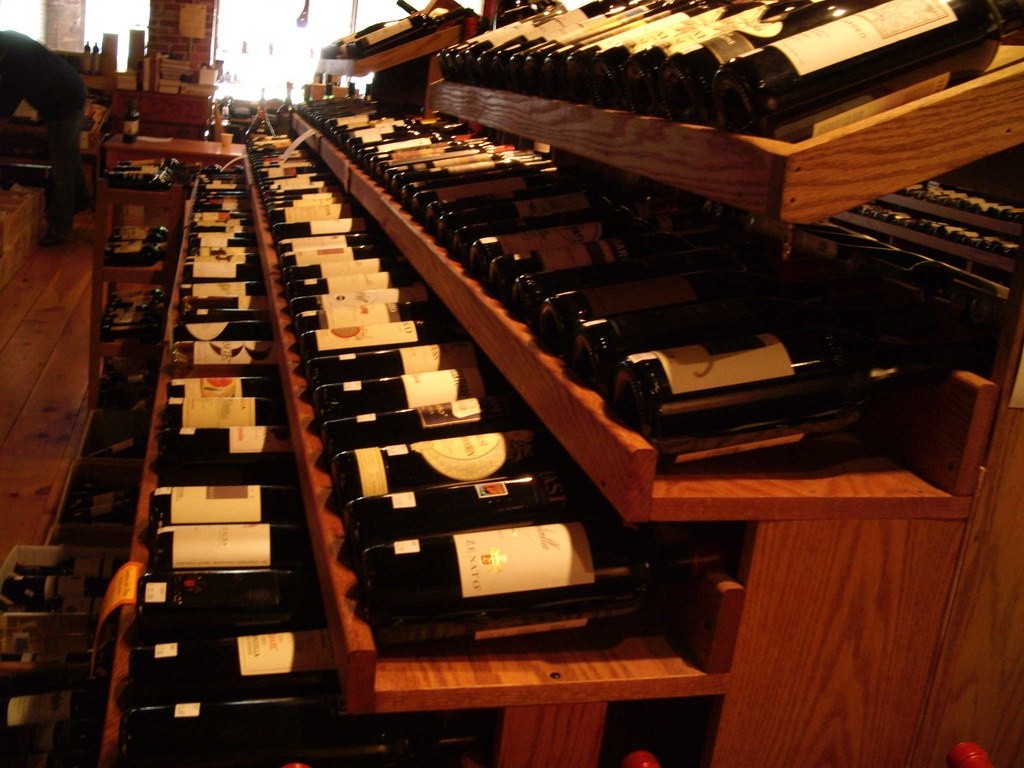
[100,1,1023,768]
[87,177,187,411]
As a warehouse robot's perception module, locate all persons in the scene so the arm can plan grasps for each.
[0,33,87,247]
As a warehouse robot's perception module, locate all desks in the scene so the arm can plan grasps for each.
[103,135,245,169]
[109,87,218,140]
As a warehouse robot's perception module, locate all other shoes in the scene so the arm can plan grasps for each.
[71,193,92,215]
[39,232,67,246]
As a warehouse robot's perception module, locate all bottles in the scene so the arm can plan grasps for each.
[0,0,1024,768]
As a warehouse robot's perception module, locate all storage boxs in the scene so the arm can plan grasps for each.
[0,411,155,768]
